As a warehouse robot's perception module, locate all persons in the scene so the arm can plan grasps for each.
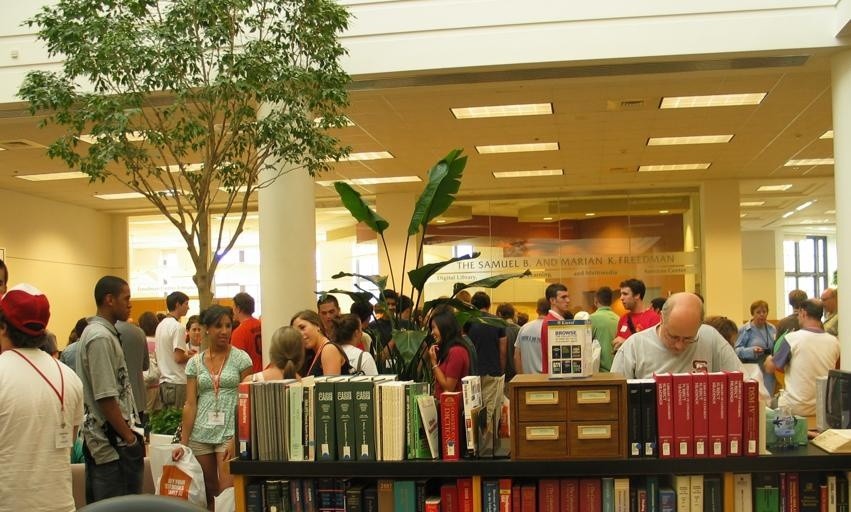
[0,259,520,407]
[174,305,255,512]
[1,285,84,511]
[610,292,748,376]
[514,279,666,376]
[77,277,147,502]
[734,289,840,431]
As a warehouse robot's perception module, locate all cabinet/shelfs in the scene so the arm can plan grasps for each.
[509,373,629,461]
[223,441,851,512]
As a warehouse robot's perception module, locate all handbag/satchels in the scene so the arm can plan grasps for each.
[143,352,160,383]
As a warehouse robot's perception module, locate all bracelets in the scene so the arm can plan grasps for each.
[127,435,137,447]
[178,439,188,446]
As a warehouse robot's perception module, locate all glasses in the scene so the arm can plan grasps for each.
[668,334,699,343]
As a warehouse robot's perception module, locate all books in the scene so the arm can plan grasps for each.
[626,373,766,457]
[233,373,850,511]
[544,319,594,380]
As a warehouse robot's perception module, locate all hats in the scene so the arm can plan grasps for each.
[0,284,50,336]
